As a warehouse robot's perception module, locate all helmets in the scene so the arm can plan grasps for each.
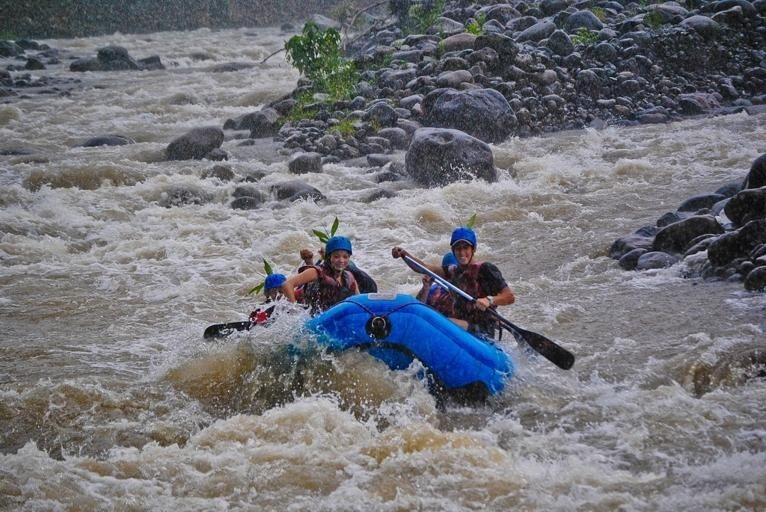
[442,252,459,266]
[264,273,287,288]
[451,227,477,247]
[326,235,353,254]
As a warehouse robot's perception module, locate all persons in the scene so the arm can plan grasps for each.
[281,235,360,319]
[298,246,326,319]
[391,226,515,345]
[247,248,314,330]
[416,251,459,319]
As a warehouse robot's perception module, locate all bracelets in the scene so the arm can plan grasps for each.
[487,294,495,307]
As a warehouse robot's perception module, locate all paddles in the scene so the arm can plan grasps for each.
[406,257,575,369]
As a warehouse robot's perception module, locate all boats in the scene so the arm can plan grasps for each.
[283,292,517,407]
